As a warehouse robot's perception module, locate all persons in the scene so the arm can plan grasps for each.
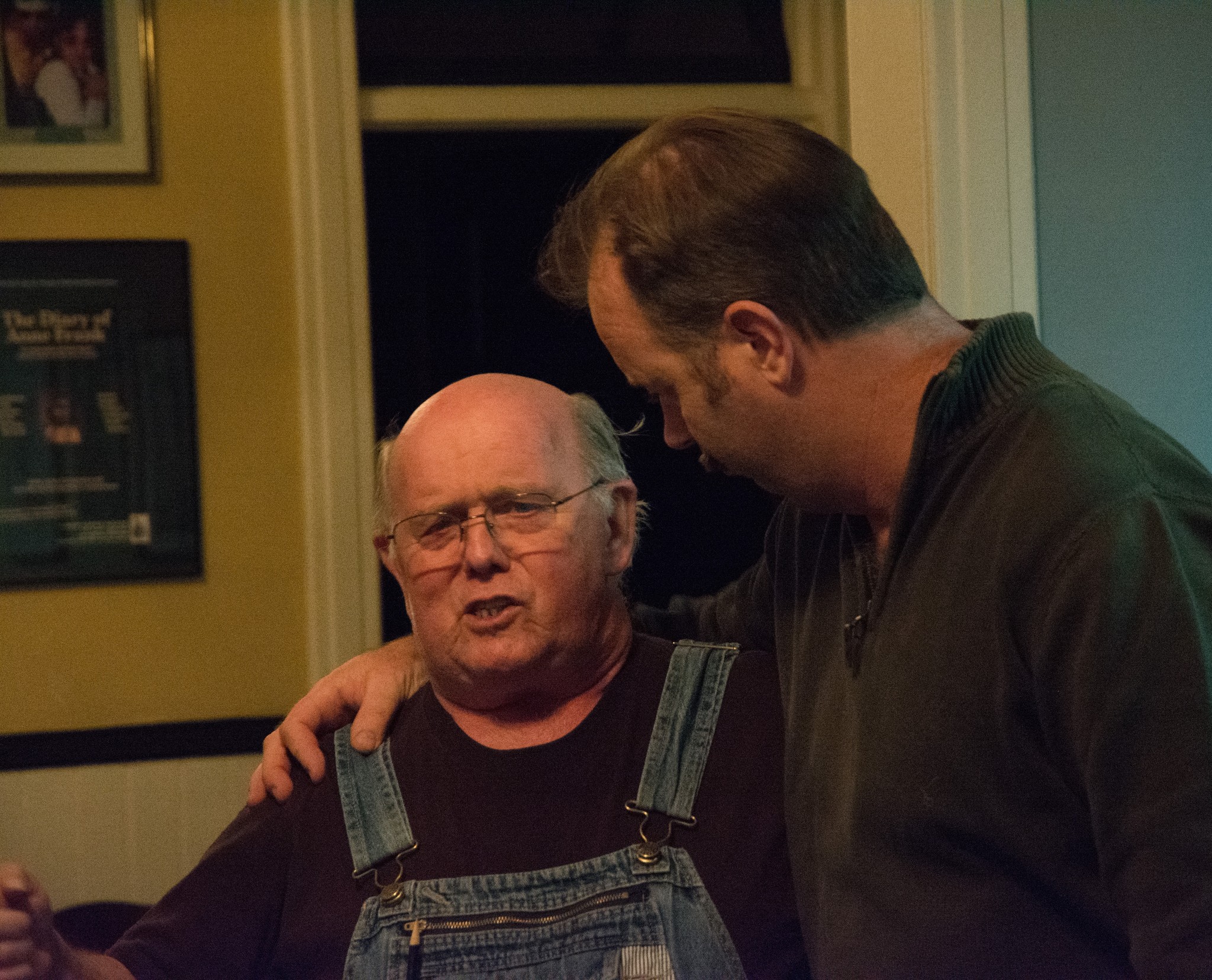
[35,18,107,126]
[248,111,1212,979]
[0,373,811,978]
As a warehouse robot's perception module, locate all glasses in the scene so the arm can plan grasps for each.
[386,478,607,574]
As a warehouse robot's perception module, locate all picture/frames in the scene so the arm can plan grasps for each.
[0,0,161,187]
